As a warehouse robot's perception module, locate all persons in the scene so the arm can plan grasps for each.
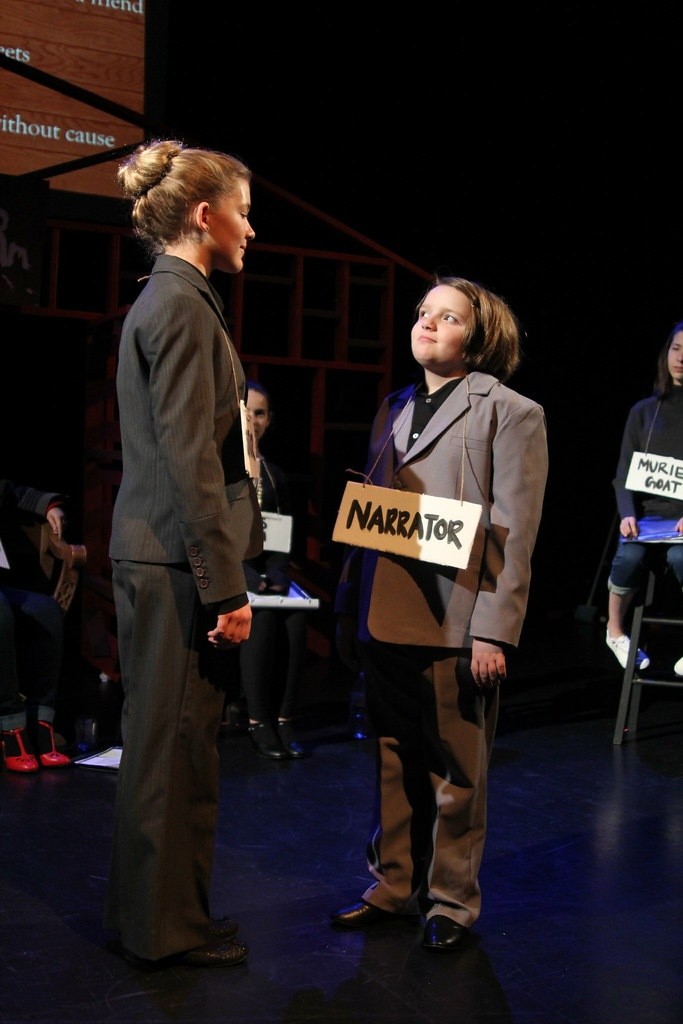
[329,273,549,948]
[223,379,312,762]
[109,135,257,975]
[601,324,683,680]
[0,478,73,773]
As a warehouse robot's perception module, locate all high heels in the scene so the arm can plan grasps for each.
[38,721,71,766]
[1,726,39,771]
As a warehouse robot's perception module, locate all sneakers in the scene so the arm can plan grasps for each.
[606,621,650,670]
[674,656,683,676]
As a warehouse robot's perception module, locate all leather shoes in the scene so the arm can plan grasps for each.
[122,915,249,968]
[330,887,395,928]
[277,722,309,758]
[248,720,286,759]
[423,911,468,950]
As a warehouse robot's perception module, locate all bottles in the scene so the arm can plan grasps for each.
[349,672,369,739]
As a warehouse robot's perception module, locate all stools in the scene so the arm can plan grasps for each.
[613,546,683,745]
